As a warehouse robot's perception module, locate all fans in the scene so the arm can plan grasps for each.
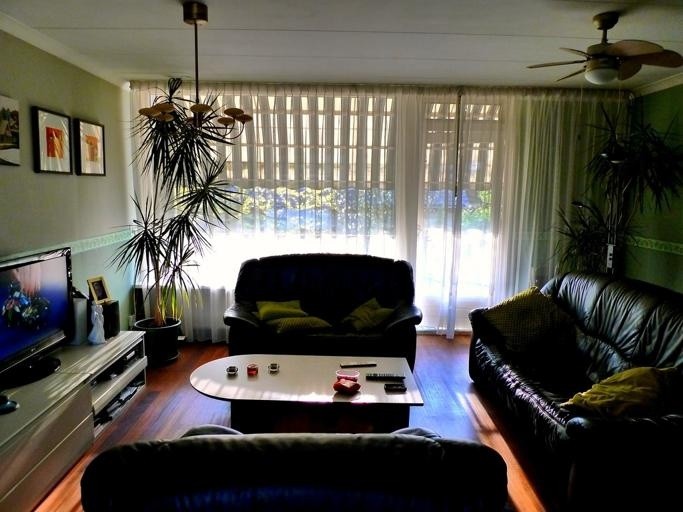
[526,12,682,85]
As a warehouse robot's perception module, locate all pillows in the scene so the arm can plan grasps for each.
[267,317,332,334]
[342,297,395,332]
[562,366,677,417]
[482,286,570,354]
[255,300,309,321]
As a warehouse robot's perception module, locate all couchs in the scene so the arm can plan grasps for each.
[79,425,507,512]
[468,271,682,511]
[222,254,423,373]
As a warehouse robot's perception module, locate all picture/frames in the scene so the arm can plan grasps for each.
[73,118,105,176]
[0,95,21,166]
[87,276,111,305]
[30,106,73,175]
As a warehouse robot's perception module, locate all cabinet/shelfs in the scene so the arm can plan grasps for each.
[1,330,148,510]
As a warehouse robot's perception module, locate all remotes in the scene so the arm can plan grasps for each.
[338,361,382,369]
[365,371,407,382]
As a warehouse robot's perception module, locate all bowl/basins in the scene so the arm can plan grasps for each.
[335,369,360,380]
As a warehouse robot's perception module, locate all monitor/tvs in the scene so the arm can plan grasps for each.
[0,245,77,394]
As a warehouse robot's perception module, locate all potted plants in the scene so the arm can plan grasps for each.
[108,78,247,369]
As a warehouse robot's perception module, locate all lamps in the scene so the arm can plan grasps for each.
[138,0,253,139]
[585,59,618,85]
